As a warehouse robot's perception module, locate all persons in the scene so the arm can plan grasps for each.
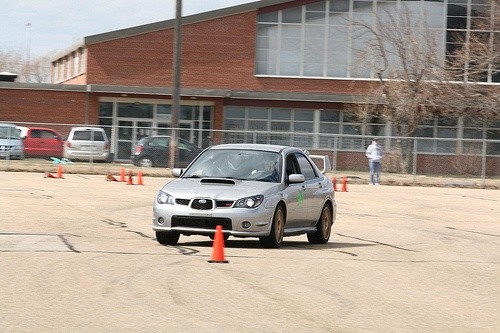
[251,158,288,180]
[215,153,248,177]
[366,138,384,185]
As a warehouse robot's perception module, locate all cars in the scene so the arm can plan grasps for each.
[22,127,64,160]
[130,134,203,169]
[151,143,338,250]
[16,126,30,138]
[0,124,28,162]
[64,125,110,162]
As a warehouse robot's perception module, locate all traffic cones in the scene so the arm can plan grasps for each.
[106,173,119,181]
[135,169,144,186]
[332,177,337,192]
[127,169,136,186]
[120,166,128,182]
[341,175,348,192]
[56,165,64,180]
[44,170,56,179]
[207,225,230,264]
